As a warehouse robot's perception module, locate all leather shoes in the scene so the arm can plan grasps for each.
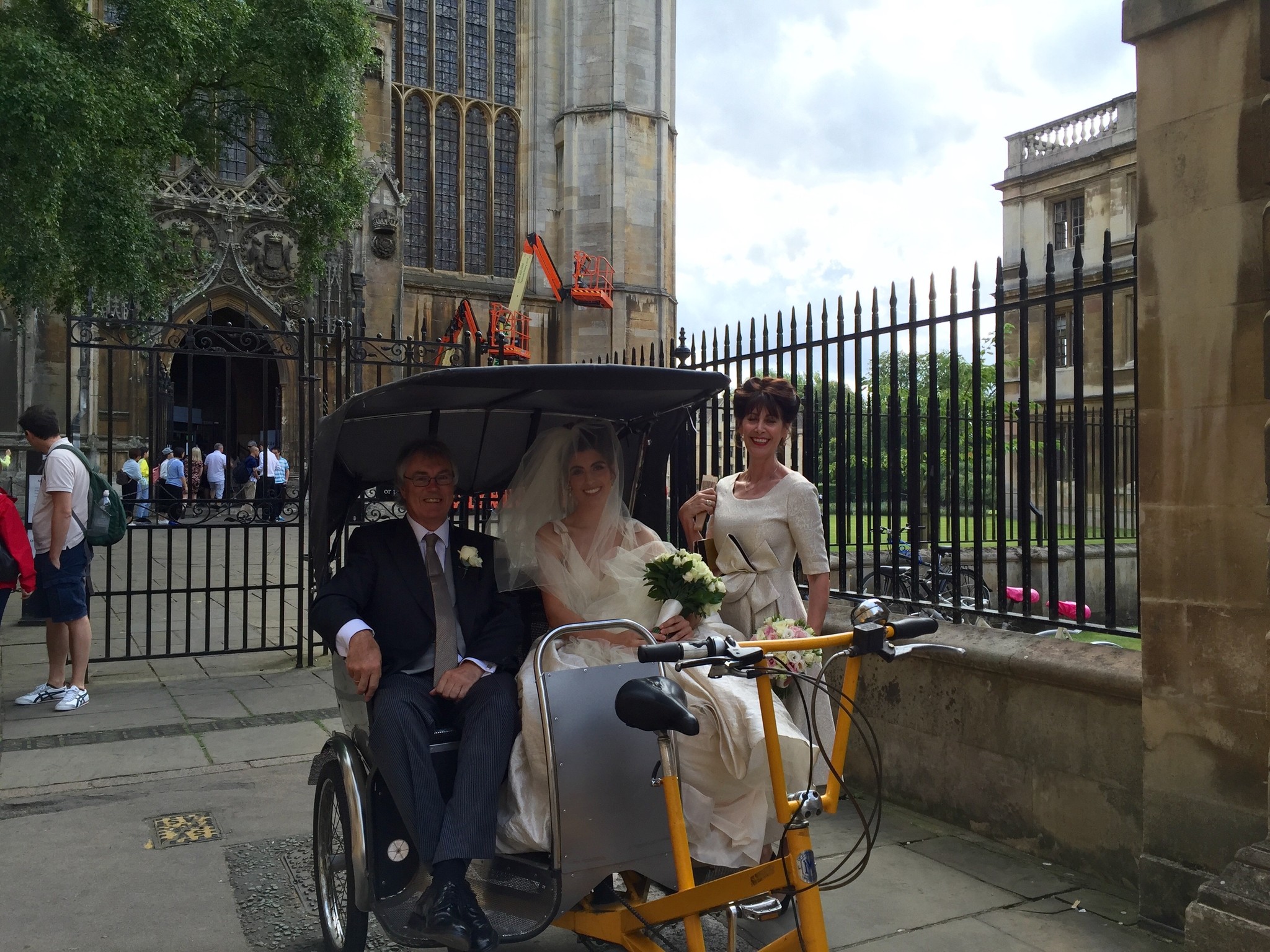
[405,882,472,952]
[446,880,499,952]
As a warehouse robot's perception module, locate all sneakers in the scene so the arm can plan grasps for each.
[276,515,285,521]
[15,682,67,704]
[55,686,90,710]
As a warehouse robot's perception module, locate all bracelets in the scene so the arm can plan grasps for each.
[285,480,287,482]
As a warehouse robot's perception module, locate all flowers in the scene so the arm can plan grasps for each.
[751,614,822,700]
[458,545,483,579]
[642,548,727,641]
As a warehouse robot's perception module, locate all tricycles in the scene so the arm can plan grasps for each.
[299,359,946,951]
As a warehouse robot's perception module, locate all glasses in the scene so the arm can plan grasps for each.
[272,452,279,454]
[247,445,254,448]
[403,474,455,487]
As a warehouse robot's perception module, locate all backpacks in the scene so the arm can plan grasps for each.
[232,458,255,484]
[116,471,127,485]
[153,464,160,484]
[43,445,126,546]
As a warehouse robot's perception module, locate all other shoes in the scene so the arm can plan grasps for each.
[215,512,221,517]
[255,518,269,523]
[246,517,252,521]
[158,519,169,524]
[238,516,247,521]
[180,509,185,519]
[127,523,137,525]
[193,515,198,517]
[142,522,149,525]
[168,521,180,525]
[135,522,142,525]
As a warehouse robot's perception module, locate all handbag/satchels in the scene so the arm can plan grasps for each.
[0,538,20,582]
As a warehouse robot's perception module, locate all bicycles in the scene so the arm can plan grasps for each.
[865,523,1092,620]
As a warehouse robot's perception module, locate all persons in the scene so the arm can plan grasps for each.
[230,444,240,499]
[155,447,188,524]
[486,417,819,952]
[14,404,94,710]
[122,447,152,526]
[0,460,36,625]
[248,440,259,466]
[238,446,260,521]
[308,440,527,952]
[0,449,11,467]
[271,446,290,522]
[203,440,233,517]
[254,441,281,522]
[680,376,844,901]
[181,447,203,519]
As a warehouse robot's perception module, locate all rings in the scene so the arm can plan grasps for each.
[708,500,712,506]
[706,499,709,505]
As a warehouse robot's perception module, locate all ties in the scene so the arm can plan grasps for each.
[422,532,458,688]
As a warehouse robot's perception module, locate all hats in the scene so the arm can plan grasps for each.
[162,447,173,455]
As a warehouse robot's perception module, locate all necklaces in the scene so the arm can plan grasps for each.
[745,461,779,493]
